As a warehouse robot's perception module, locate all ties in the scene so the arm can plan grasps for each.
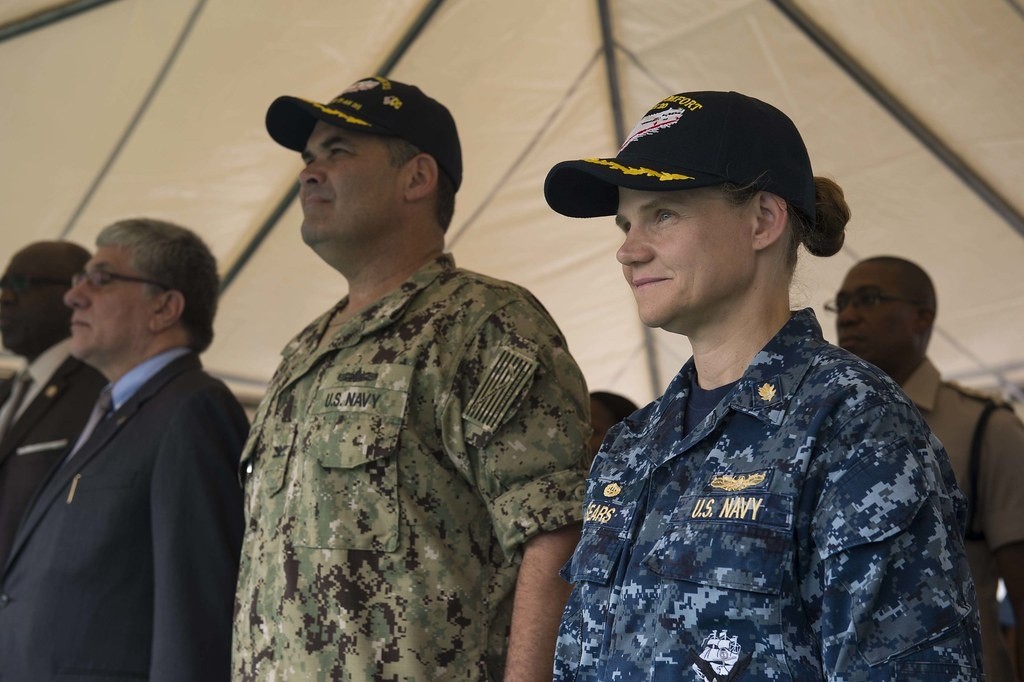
[3,370,35,436]
[64,387,114,463]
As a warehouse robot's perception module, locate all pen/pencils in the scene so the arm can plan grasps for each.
[66,474,81,503]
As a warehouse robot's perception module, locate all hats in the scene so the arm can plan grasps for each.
[542,90,818,228]
[264,77,463,193]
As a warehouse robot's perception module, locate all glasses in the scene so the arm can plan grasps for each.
[822,287,925,311]
[2,273,69,291]
[70,266,174,290]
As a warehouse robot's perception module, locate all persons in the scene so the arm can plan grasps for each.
[544,91,984,682]
[590,391,638,452]
[230,77,594,682]
[0,219,250,682]
[825,257,1024,682]
[0,239,108,575]
